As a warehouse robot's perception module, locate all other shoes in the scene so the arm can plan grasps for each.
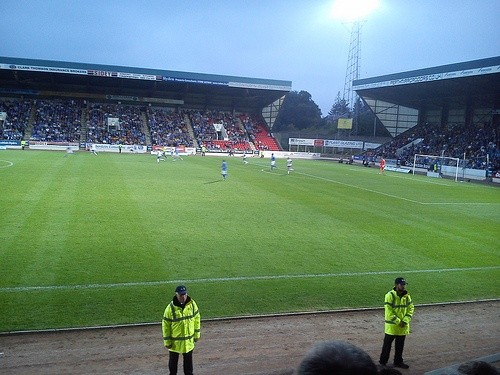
[394,362,409,369]
[380,363,386,367]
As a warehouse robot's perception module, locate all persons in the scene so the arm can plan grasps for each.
[379,278,414,368]
[0,97,293,181]
[296,340,500,375]
[348,121,500,177]
[162,286,201,375]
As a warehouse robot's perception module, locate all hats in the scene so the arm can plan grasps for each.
[395,278,408,285]
[174,285,187,294]
